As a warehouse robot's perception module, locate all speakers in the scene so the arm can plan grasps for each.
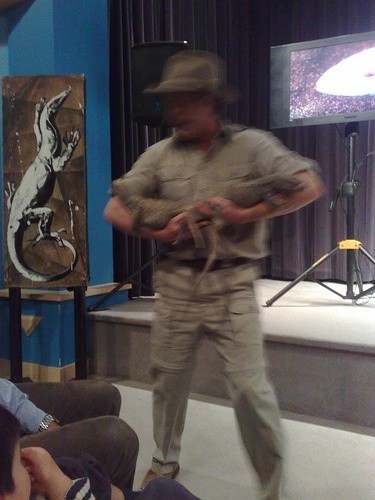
[130,40,189,128]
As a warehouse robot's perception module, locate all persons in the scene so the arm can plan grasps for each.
[104,50,325,500]
[0,377,205,499]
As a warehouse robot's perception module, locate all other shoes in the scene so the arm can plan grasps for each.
[142,465,180,487]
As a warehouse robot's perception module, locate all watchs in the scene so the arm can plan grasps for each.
[37,414,60,432]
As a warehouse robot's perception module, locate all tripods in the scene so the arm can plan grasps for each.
[259,122,375,307]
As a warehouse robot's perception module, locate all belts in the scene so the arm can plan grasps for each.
[178,257,248,273]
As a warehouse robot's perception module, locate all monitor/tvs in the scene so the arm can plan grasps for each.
[270,31,375,129]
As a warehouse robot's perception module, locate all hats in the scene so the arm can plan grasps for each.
[143,50,238,102]
